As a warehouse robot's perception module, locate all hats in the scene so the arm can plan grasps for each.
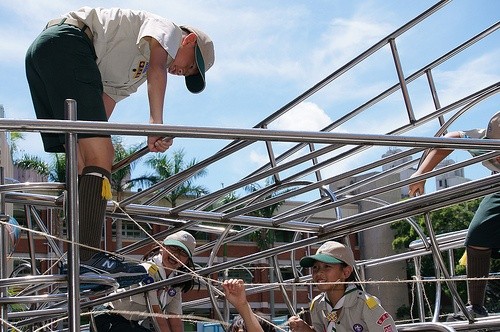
[162,230,197,257]
[299,240,355,268]
[485,112,500,139]
[180,24,215,95]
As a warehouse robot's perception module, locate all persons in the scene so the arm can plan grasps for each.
[406,111,500,319]
[88,231,197,332]
[287,241,399,332]
[221,278,279,332]
[23,7,216,292]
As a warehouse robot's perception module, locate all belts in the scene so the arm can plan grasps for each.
[47,17,94,43]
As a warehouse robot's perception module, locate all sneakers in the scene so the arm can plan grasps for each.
[57,251,150,292]
[453,305,489,321]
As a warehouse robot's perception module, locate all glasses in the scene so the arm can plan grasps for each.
[167,245,189,257]
